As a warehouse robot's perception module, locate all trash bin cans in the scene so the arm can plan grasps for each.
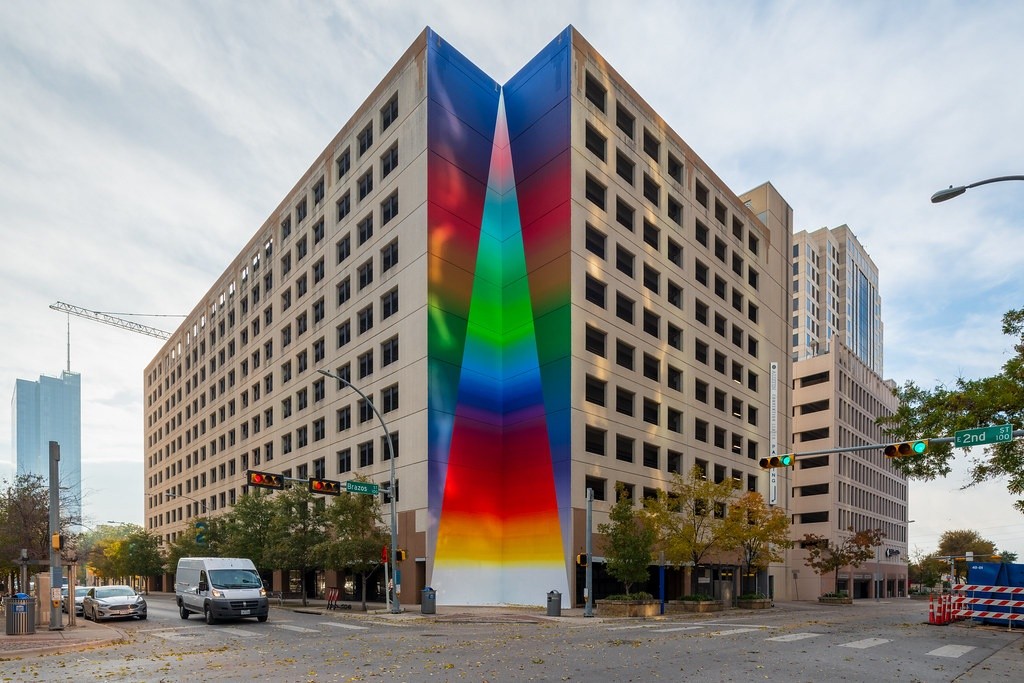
[547,590,562,615]
[420,587,437,614]
[3,593,37,635]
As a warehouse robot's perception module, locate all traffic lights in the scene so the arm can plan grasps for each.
[51,532,64,550]
[308,478,340,496]
[759,453,795,469]
[576,553,587,566]
[883,439,929,459]
[396,549,405,562]
[246,469,284,490]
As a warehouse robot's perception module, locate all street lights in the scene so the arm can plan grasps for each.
[317,369,400,614]
[165,493,211,548]
[877,520,915,602]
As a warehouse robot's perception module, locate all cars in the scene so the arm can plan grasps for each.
[82,585,147,623]
[61,584,92,616]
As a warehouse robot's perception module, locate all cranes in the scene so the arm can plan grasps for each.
[49,301,187,370]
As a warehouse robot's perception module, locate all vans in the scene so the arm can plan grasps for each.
[176,557,268,625]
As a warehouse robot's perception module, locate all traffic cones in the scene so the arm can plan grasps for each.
[923,593,971,626]
